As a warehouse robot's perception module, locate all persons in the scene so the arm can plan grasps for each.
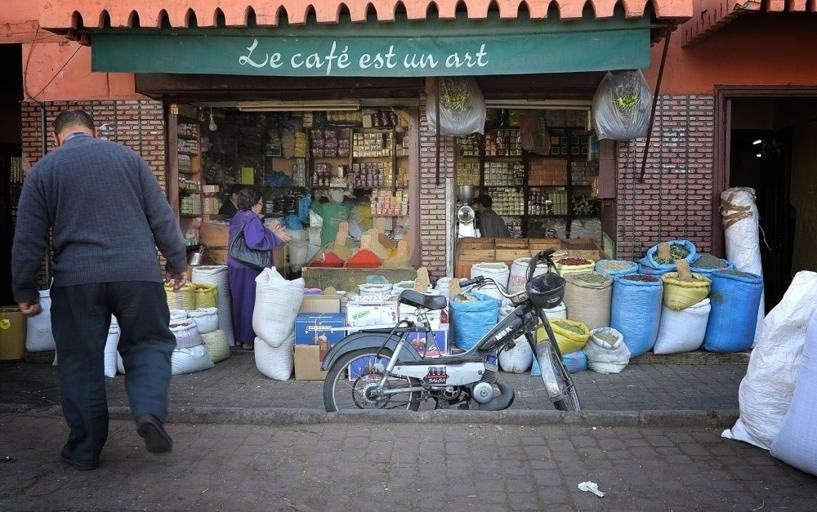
[11,109,188,471]
[477,195,510,238]
[227,187,292,351]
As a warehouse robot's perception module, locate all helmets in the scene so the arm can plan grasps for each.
[525,272,566,309]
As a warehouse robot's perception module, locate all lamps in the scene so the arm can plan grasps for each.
[209,103,217,131]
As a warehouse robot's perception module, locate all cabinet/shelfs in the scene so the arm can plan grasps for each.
[178,102,411,264]
[456,109,602,270]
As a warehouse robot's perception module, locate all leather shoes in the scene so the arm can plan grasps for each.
[136,413,174,453]
[60,443,97,471]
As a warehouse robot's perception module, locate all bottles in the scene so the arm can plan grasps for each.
[176,123,200,214]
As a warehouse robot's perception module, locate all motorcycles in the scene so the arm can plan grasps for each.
[322,247,582,413]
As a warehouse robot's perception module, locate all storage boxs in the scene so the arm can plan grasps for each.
[292,289,447,382]
[0,307,28,360]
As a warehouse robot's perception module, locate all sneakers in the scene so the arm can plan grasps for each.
[235,340,254,350]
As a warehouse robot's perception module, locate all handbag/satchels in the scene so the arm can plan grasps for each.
[229,212,272,272]
